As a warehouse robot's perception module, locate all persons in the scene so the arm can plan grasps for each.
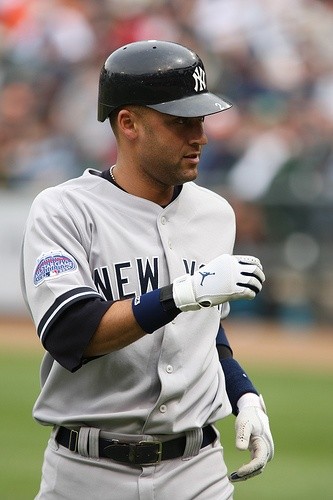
[19,40,275,500]
[0,1,333,317]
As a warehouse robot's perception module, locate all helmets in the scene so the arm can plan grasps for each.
[98,39,233,123]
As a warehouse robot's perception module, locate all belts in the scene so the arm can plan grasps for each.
[56,428,219,463]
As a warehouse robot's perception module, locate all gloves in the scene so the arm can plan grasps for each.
[171,253,266,313]
[228,393,274,482]
